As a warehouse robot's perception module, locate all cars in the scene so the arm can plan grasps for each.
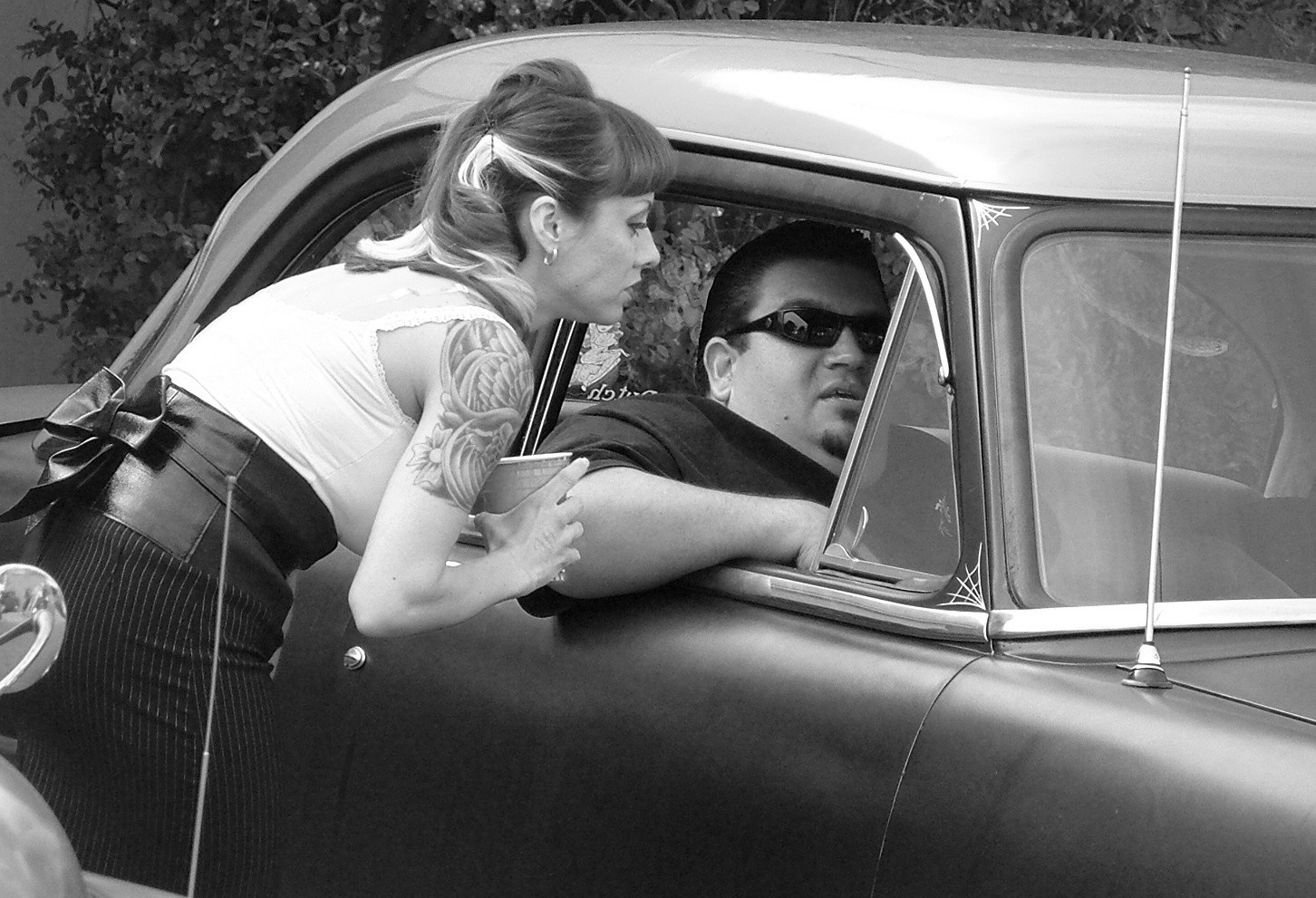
[0,15,1315,898]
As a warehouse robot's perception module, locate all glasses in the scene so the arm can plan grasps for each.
[724,306,886,355]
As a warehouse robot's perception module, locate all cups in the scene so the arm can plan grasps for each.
[485,453,573,517]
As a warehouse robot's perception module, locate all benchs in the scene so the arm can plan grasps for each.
[888,425,1316,605]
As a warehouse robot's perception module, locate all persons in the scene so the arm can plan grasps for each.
[0,59,678,898]
[516,220,958,619]
